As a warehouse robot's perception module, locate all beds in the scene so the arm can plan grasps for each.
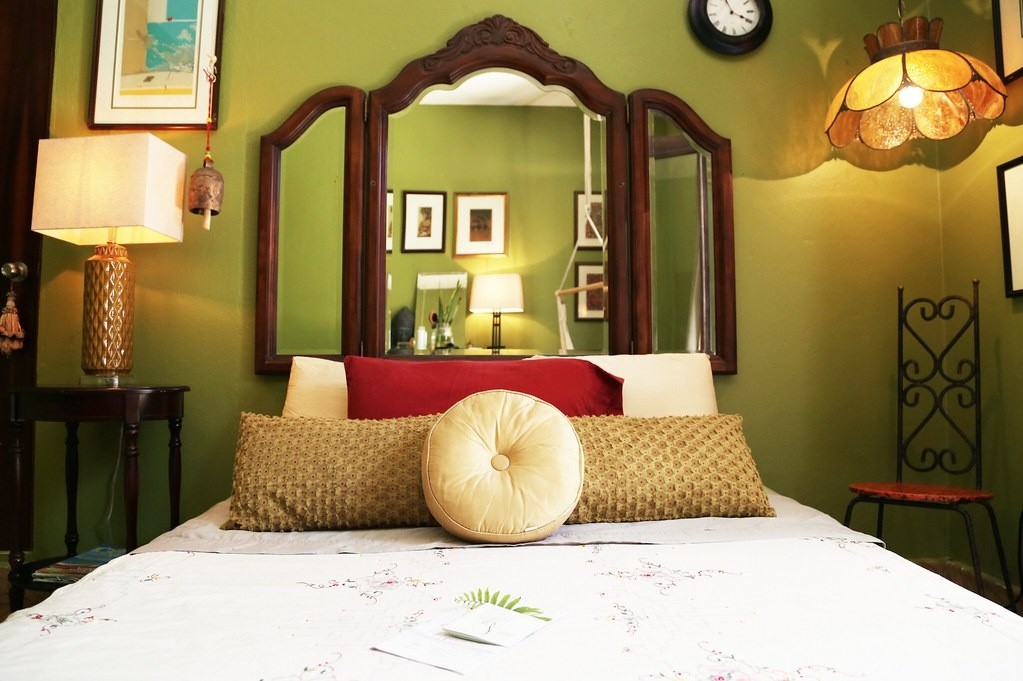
[0,354,1023,680]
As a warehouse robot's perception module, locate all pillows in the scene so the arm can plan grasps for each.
[218,352,778,544]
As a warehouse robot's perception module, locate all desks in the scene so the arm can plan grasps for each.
[0,383,190,616]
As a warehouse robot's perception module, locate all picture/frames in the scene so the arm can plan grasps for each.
[996,155,1023,298]
[382,189,510,258]
[86,0,226,131]
[573,190,607,251]
[992,0,1023,85]
[574,261,605,323]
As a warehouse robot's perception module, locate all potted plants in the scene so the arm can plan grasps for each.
[431,279,464,352]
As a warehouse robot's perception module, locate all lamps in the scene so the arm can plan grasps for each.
[469,273,524,354]
[30,133,186,388]
[825,0,1008,150]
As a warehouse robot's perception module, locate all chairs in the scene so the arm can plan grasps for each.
[842,278,1018,615]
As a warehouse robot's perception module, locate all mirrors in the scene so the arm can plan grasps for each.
[251,14,737,376]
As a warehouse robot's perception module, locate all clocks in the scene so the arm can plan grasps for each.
[687,0,773,55]
